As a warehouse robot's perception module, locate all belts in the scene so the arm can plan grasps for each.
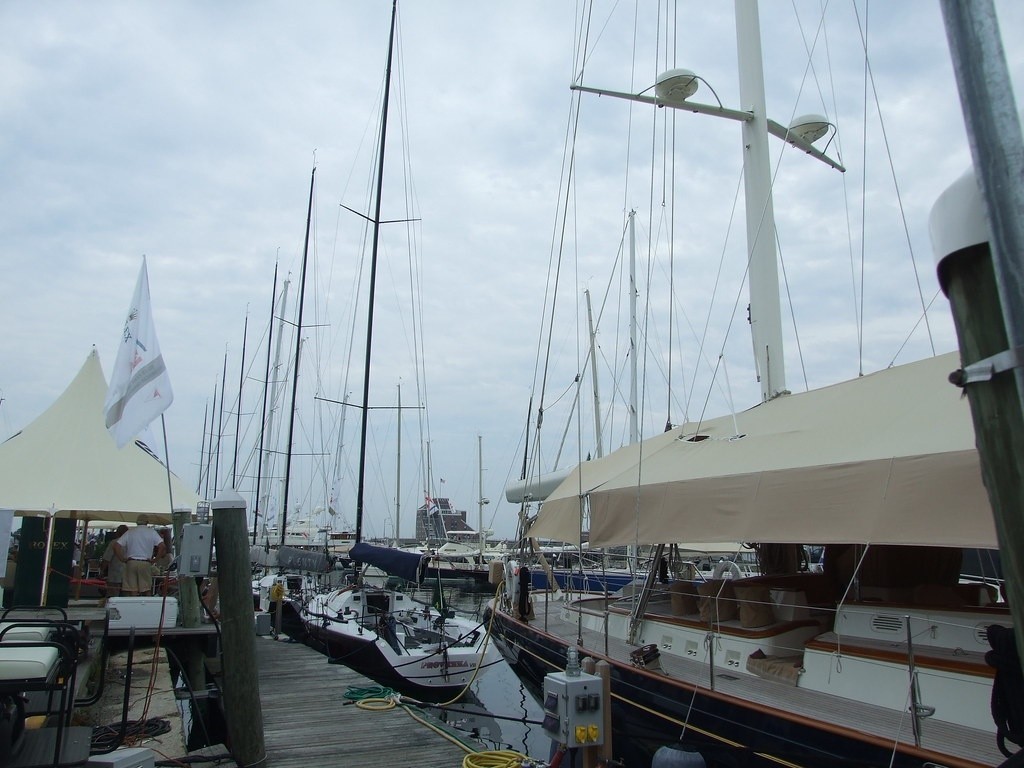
[129,558,149,561]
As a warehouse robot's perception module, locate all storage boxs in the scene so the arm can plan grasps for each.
[103,597,179,629]
[88,747,155,768]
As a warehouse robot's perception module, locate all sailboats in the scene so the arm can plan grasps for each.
[193,0,761,707]
[477,0,1024,768]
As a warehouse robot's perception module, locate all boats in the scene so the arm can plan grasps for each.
[248,506,356,552]
[415,498,589,546]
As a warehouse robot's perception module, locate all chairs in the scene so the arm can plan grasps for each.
[85,558,101,581]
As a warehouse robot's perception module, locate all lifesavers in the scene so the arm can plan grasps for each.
[505,560,521,612]
[712,560,742,580]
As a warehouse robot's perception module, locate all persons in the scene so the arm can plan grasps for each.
[11,544,18,558]
[17,534,21,540]
[115,513,165,596]
[101,525,129,598]
[760,543,801,574]
[73,528,115,578]
[152,548,173,570]
[804,551,820,563]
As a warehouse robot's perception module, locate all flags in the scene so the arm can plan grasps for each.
[441,478,446,483]
[103,260,173,448]
[330,479,341,515]
[425,492,438,516]
